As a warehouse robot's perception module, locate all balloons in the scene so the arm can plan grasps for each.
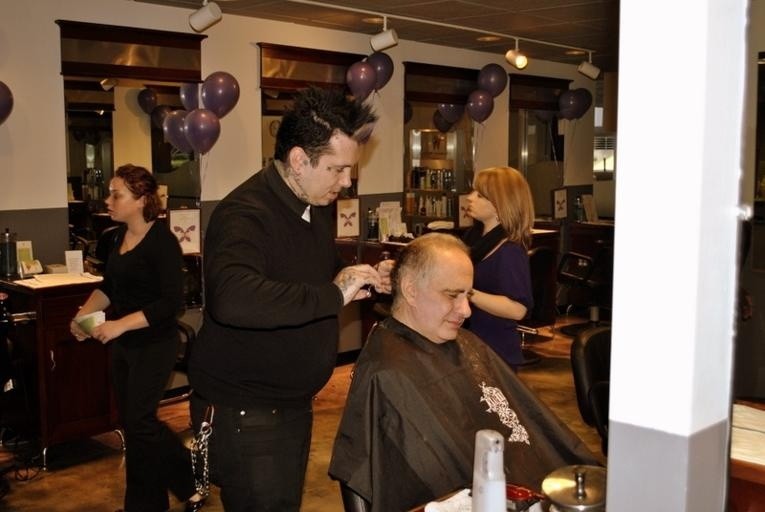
[137,88,157,114]
[478,63,508,97]
[432,110,455,133]
[152,105,171,129]
[184,109,220,156]
[367,52,394,92]
[346,61,376,100]
[438,103,465,124]
[467,90,494,125]
[201,71,240,119]
[558,89,584,121]
[162,110,193,154]
[405,102,412,124]
[574,88,592,120]
[180,83,198,111]
[0,81,13,126]
[533,109,552,123]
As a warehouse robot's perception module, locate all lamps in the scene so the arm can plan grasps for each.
[188,1,600,81]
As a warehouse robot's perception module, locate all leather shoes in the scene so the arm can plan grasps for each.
[186,495,206,511]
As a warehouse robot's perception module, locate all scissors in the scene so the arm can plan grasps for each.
[365,261,381,297]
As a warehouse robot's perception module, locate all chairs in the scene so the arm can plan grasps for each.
[569,324,611,460]
[338,434,373,512]
[508,243,563,368]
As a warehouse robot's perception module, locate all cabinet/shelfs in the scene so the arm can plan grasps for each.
[0,271,127,472]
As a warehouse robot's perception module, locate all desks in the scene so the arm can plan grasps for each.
[356,228,563,352]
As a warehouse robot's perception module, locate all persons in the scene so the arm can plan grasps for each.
[183,84,398,512]
[69,162,208,512]
[461,166,535,377]
[326,232,606,511]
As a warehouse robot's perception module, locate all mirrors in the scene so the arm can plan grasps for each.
[54,17,208,272]
[400,58,476,239]
[255,40,360,240]
[506,72,575,222]
